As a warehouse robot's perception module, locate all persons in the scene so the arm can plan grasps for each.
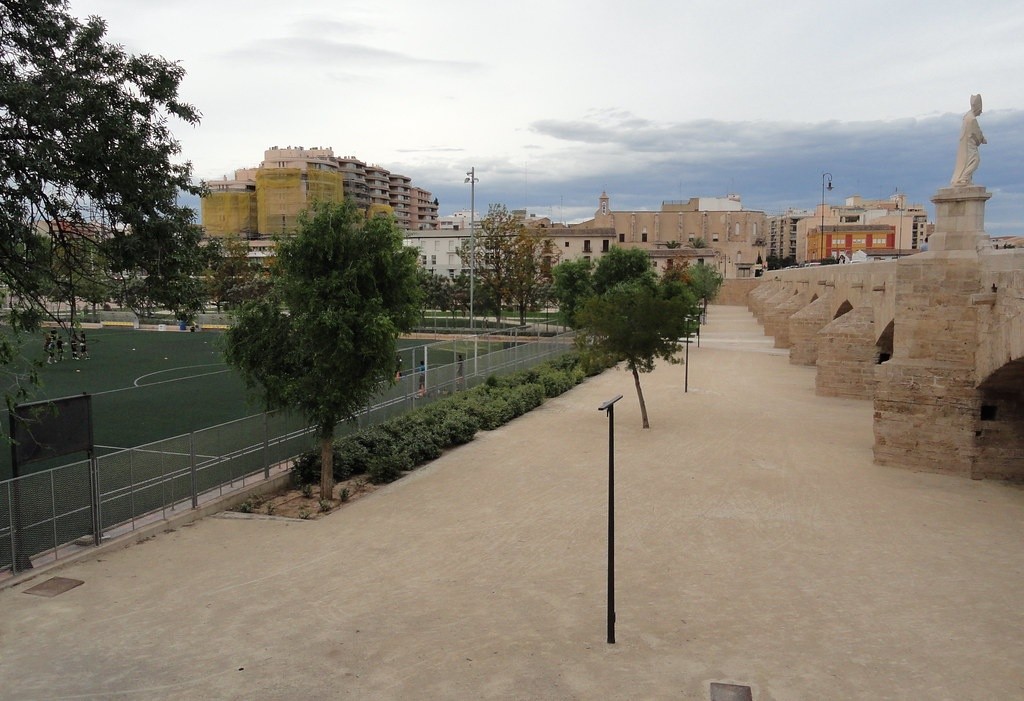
[919,237,929,252]
[417,360,428,394]
[454,354,465,394]
[43,327,91,365]
[393,352,404,378]
[837,254,849,264]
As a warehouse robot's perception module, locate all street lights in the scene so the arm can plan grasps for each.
[720,254,731,278]
[820,173,835,263]
[895,197,903,259]
[464,167,479,330]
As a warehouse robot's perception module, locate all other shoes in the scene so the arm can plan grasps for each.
[72,358,74,360]
[47,361,52,364]
[76,358,79,360]
[54,360,58,363]
[86,357,90,360]
[79,356,82,358]
[59,360,61,361]
[64,359,67,360]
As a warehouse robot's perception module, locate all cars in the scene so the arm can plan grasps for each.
[785,263,821,269]
